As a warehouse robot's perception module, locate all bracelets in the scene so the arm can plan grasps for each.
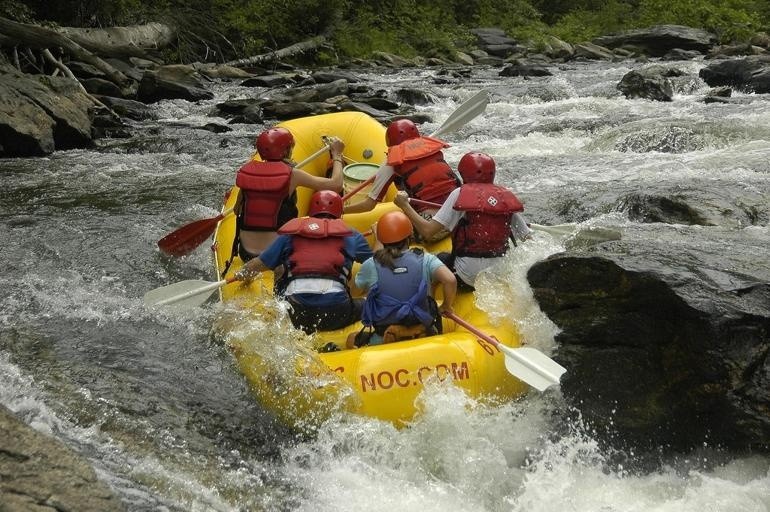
[333,157,345,165]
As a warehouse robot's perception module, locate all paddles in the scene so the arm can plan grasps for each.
[143,231,372,307]
[444,311,567,392]
[157,144,331,260]
[408,198,621,245]
[340,88,490,200]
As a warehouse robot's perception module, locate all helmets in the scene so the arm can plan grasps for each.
[256,126,294,160]
[457,151,496,184]
[385,118,420,147]
[376,210,413,244]
[308,190,344,218]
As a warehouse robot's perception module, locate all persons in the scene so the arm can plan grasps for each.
[232,125,347,265]
[340,119,461,244]
[342,208,459,349]
[391,149,534,294]
[239,188,386,334]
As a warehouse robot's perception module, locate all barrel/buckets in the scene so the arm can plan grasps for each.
[341,162,386,206]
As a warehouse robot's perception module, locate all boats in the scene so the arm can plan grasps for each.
[210,111,530,433]
